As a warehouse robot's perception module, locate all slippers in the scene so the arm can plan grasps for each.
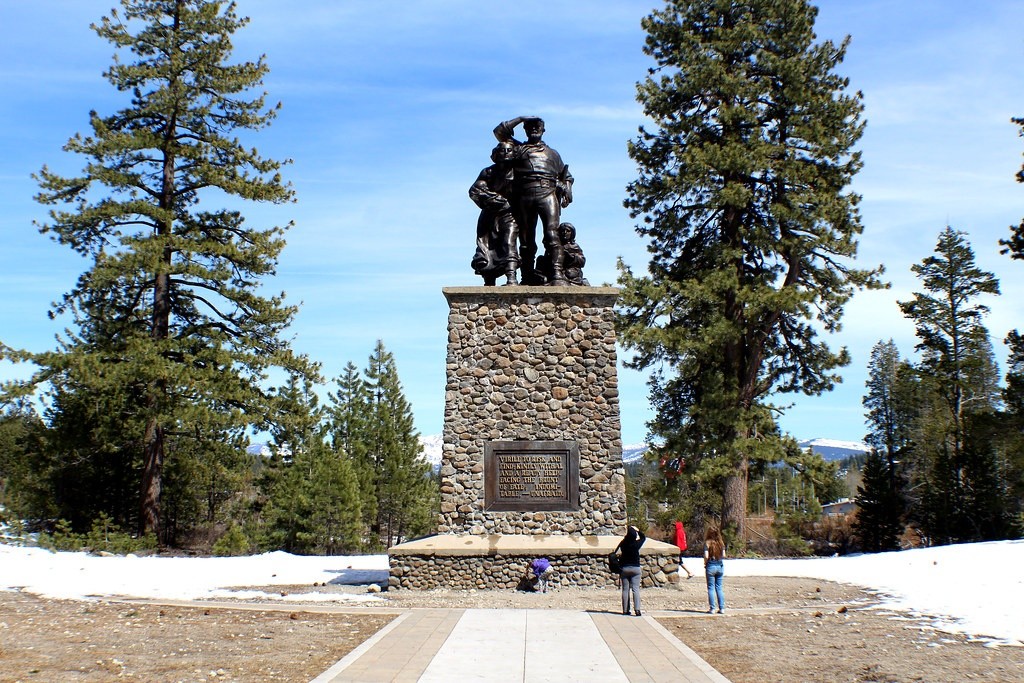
[687,574,694,579]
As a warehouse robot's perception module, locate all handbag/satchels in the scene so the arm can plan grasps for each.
[609,552,621,574]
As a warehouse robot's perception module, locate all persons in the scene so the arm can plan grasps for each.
[618,525,646,615]
[670,522,695,580]
[702,526,728,614]
[528,558,554,593]
[468,115,589,286]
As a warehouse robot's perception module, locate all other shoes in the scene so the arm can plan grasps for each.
[718,609,725,614]
[635,610,641,616]
[707,609,715,613]
[623,611,631,615]
